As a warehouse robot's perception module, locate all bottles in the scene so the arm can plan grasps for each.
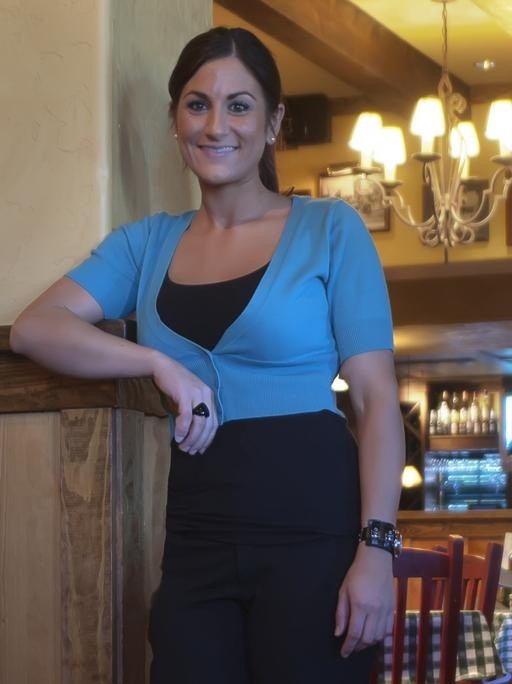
[429,387,496,436]
[448,504,469,512]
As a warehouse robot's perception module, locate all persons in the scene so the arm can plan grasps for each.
[10,26,406,684]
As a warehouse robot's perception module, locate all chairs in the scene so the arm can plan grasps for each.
[367,533,464,683]
[430,541,503,628]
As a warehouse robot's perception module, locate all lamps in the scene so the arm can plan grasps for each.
[347,0,512,251]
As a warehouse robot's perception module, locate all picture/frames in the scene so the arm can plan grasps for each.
[503,169,512,247]
[422,179,489,242]
[317,170,390,232]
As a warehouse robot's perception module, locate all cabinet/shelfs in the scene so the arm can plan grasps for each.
[420,376,506,451]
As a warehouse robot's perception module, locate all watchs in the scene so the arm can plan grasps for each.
[357,519,403,560]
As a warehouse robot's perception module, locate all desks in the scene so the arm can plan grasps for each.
[376,607,506,684]
[492,611,512,684]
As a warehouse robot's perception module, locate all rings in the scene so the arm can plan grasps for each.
[192,402,209,417]
